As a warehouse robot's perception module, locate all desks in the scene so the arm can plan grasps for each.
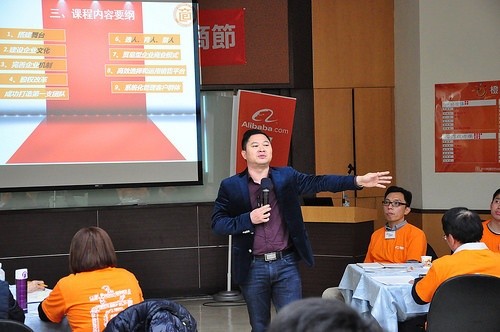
[338,262,430,332]
[8,285,73,332]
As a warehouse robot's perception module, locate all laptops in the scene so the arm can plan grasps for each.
[303,197,333,206]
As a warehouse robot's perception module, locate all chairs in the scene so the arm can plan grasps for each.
[426,274,500,332]
[102,299,199,332]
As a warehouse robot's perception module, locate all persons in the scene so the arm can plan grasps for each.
[411,205,500,305]
[209,129,393,332]
[0,262,45,326]
[268,298,378,332]
[363,186,427,263]
[37,225,144,332]
[478,188,500,255]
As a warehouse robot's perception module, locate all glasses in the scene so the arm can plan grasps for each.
[443,234,448,241]
[382,201,407,207]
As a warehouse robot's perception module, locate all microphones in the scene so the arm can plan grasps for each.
[261,178,272,224]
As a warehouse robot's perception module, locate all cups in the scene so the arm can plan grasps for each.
[421,255,432,265]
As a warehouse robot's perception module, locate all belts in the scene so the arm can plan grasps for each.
[252,246,296,262]
